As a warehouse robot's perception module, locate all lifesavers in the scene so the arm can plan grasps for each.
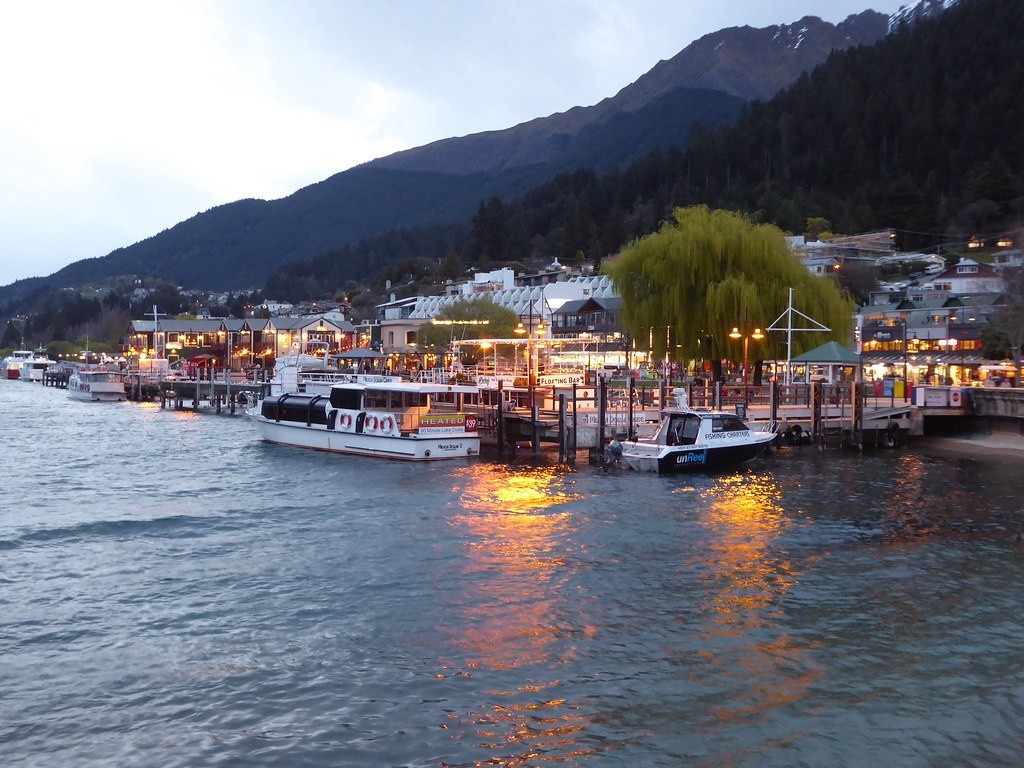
[888,422,900,438]
[340,413,352,429]
[365,414,378,432]
[380,416,393,434]
[787,425,803,439]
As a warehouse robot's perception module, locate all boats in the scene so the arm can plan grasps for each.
[0,336,33,381]
[19,341,58,381]
[606,388,779,474]
[64,334,128,402]
[244,348,483,461]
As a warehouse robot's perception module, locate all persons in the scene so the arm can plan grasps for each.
[640,362,647,380]
[945,374,953,385]
[919,373,924,382]
[985,372,1011,388]
[658,363,663,378]
[733,389,741,396]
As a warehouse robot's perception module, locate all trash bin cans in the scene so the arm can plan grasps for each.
[874,378,914,397]
[735,402,745,421]
